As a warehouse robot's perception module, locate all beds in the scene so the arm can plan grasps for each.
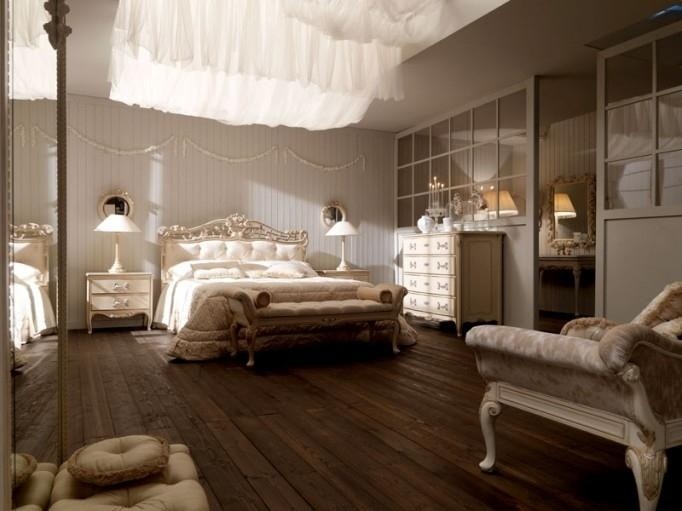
[11,225,51,365]
[158,211,373,355]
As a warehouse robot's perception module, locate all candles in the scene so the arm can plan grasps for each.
[428,175,443,209]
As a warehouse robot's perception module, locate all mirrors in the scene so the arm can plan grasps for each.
[546,173,596,251]
[9,0,67,511]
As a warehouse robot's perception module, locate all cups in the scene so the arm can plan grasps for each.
[443,218,453,232]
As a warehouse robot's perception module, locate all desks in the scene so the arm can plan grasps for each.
[539,255,596,316]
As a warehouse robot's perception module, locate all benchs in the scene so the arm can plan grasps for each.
[223,283,408,366]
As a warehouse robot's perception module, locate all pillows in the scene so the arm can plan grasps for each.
[244,287,272,308]
[167,254,320,281]
[11,259,43,283]
[65,432,173,484]
[358,284,392,303]
[10,449,38,487]
[622,281,681,327]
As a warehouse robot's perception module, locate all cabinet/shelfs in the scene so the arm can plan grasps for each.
[401,232,506,337]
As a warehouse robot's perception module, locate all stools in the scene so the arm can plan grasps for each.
[49,443,210,511]
[12,462,57,510]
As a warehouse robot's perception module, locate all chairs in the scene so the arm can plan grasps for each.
[465,281,682,511]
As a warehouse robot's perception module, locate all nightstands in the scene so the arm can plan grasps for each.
[84,271,155,337]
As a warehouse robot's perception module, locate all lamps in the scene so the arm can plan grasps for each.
[480,190,519,217]
[94,214,140,273]
[553,192,576,239]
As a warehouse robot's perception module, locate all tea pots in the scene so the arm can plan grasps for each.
[417,216,435,235]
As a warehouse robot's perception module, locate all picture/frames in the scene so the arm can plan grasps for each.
[321,199,345,230]
[98,189,134,222]
